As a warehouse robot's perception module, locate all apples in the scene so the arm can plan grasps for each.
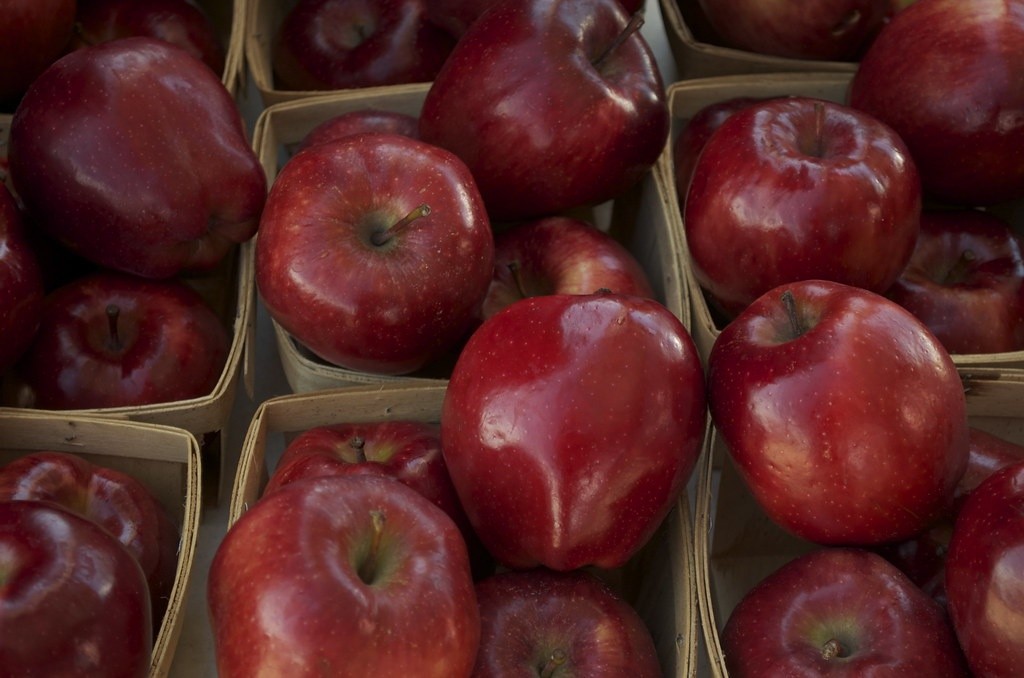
[0,0,1024,678]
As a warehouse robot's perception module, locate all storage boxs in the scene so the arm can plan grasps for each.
[0,0,1024,678]
